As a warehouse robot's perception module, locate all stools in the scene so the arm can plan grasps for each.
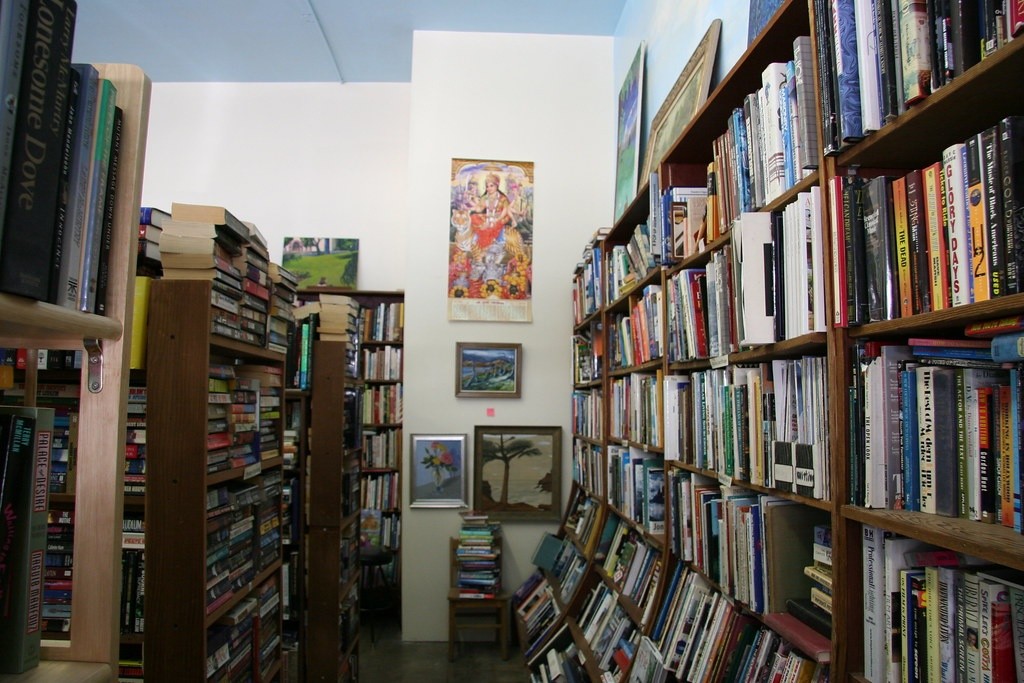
[359,545,397,653]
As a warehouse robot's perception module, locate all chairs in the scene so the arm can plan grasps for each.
[445,537,509,661]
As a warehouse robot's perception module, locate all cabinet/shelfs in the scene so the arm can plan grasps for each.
[288,287,403,623]
[0,60,152,683]
[308,341,361,683]
[514,0,1024,683]
[143,282,283,683]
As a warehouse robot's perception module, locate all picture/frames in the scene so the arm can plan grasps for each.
[411,435,469,508]
[638,17,721,196]
[455,341,523,397]
[473,425,563,522]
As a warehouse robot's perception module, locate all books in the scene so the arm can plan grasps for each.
[454,516,503,602]
[531,528,571,605]
[0,315,118,674]
[512,569,561,643]
[570,0,1024,331]
[522,640,571,683]
[571,331,1024,683]
[0,0,124,314]
[317,292,406,683]
[118,208,171,683]
[159,203,319,683]
